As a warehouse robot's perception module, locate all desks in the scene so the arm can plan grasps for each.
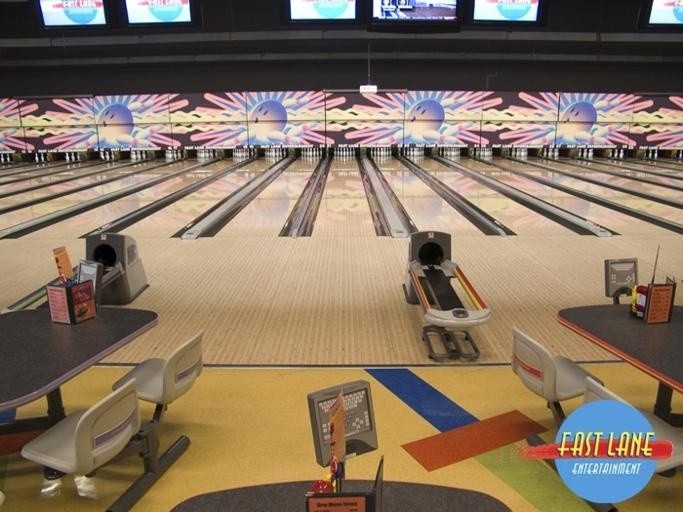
[556,303,683,478]
[0,306,158,481]
[169,480,510,512]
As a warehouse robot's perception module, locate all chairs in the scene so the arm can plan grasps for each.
[111,328,205,406]
[583,376,683,474]
[511,324,604,403]
[20,377,140,479]
